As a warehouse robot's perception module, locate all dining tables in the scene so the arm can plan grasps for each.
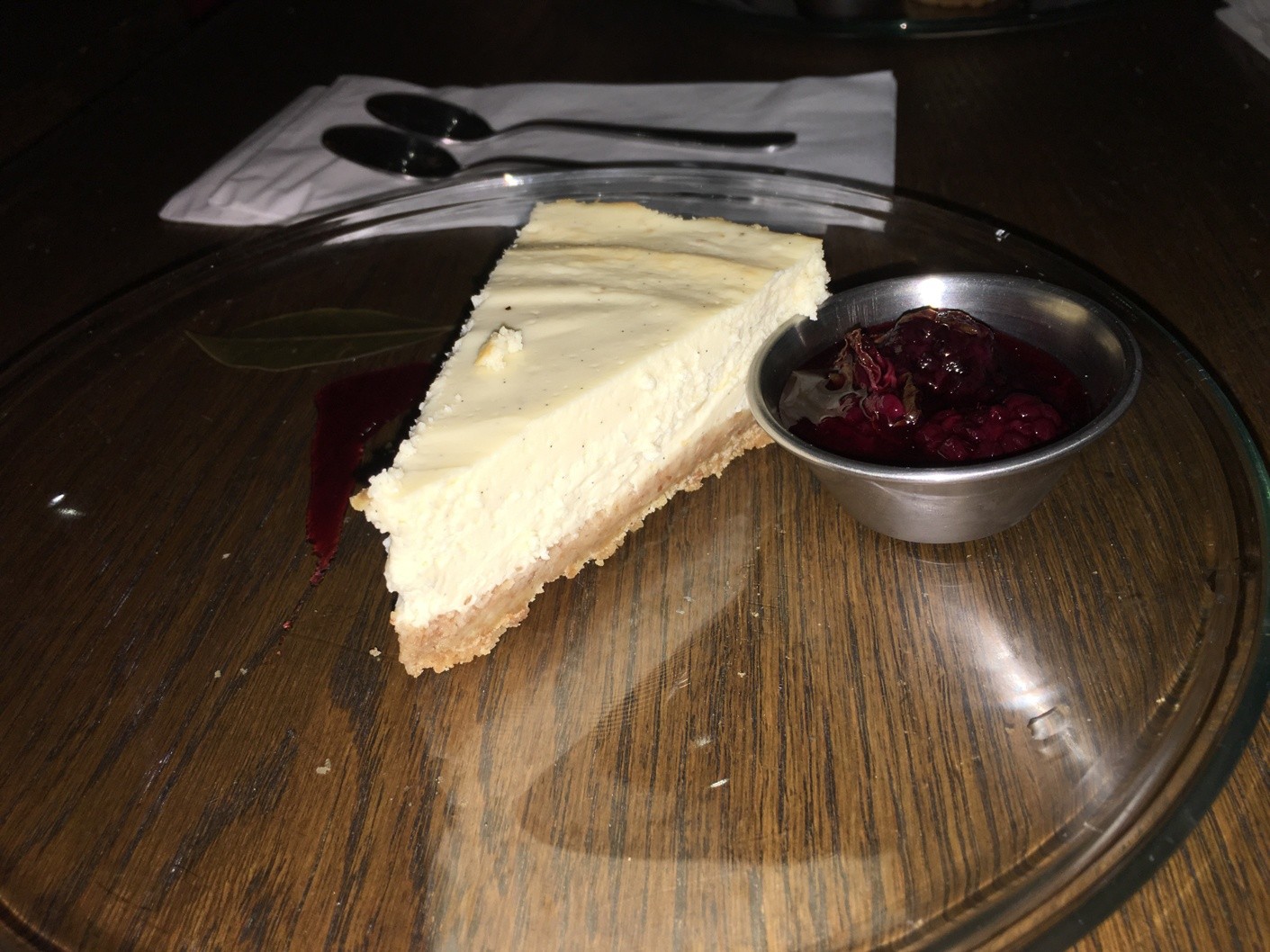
[0,0,1270,952]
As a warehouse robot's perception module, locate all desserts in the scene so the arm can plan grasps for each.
[349,202,834,675]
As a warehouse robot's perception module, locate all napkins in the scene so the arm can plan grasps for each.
[159,70,897,235]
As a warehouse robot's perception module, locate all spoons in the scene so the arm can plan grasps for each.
[362,92,799,153]
[319,124,590,184]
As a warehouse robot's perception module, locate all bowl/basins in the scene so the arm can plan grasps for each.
[746,270,1142,544]
